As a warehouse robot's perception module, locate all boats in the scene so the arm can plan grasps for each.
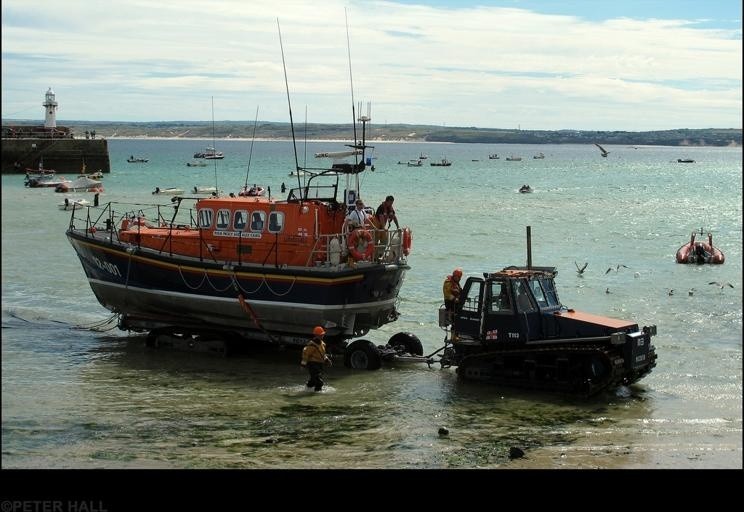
[64,8,413,346]
[125,153,150,164]
[190,145,226,161]
[188,161,211,168]
[396,148,548,169]
[191,184,221,195]
[151,185,185,196]
[24,154,73,190]
[676,156,700,164]
[519,188,533,194]
[675,230,724,264]
[59,197,92,211]
[55,158,105,193]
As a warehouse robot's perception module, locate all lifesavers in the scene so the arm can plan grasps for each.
[403,228,411,257]
[349,230,373,260]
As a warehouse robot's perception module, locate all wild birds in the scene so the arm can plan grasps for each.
[709,281,734,289]
[689,287,695,296]
[574,261,588,274]
[605,264,629,275]
[595,142,612,159]
[605,288,610,294]
[669,289,675,296]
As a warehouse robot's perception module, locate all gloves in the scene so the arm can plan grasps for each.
[325,358,332,368]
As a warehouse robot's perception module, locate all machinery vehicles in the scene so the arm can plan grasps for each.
[437,226,659,397]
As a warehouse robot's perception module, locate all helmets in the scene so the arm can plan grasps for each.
[313,326,325,335]
[454,269,462,277]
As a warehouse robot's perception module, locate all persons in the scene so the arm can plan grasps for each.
[341,199,377,265]
[373,195,400,262]
[209,183,260,199]
[516,284,529,310]
[299,325,327,391]
[442,267,467,314]
